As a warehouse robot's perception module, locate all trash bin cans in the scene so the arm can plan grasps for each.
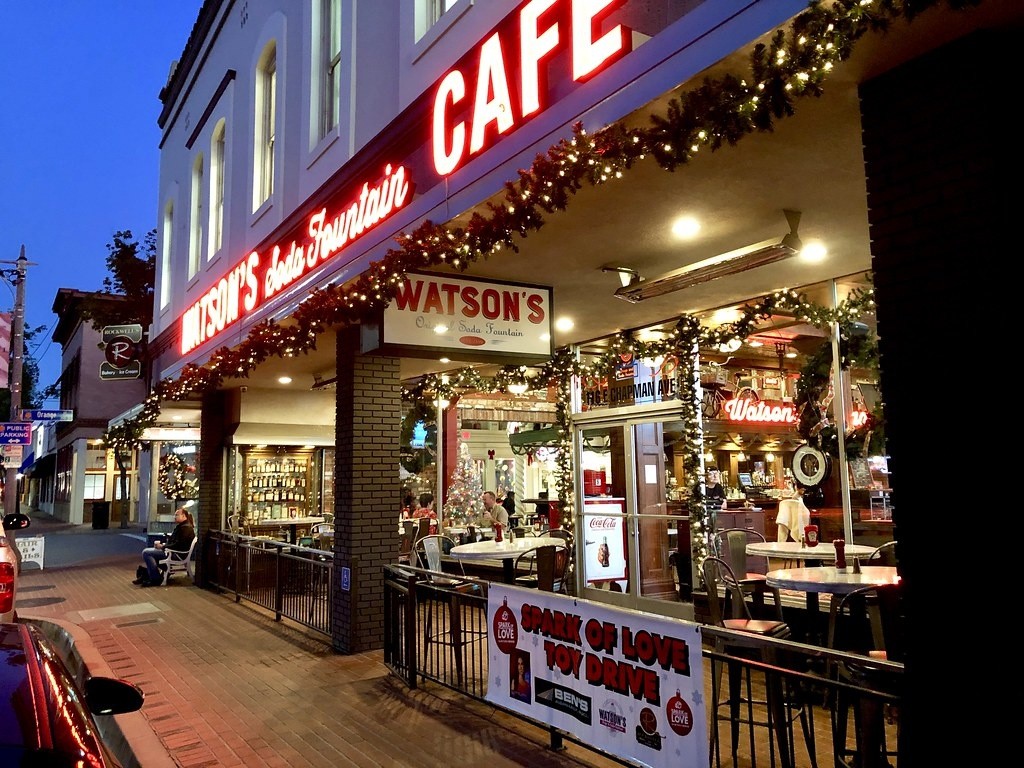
[91,501,112,530]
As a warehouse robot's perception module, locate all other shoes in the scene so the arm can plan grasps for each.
[143,578,163,587]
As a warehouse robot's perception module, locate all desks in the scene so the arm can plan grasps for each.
[261,516,325,593]
[445,526,492,534]
[765,565,898,768]
[745,541,881,705]
[450,537,566,584]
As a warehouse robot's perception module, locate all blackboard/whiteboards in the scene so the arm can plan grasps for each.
[850,449,874,488]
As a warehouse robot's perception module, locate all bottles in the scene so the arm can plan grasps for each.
[802,534,806,548]
[602,536,609,567]
[853,554,861,573]
[510,528,513,544]
[249,460,307,502]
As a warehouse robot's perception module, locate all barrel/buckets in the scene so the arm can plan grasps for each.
[584,468,607,495]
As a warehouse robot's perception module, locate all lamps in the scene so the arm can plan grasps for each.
[613,208,804,304]
[310,373,336,392]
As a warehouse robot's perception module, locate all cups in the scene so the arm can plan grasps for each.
[154,541,160,548]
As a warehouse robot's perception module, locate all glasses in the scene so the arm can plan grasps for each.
[481,499,492,502]
[708,472,720,476]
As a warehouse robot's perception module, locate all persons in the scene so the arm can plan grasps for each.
[403,495,420,517]
[412,493,437,535]
[792,482,806,500]
[131,508,194,586]
[695,466,727,509]
[476,491,508,533]
[502,491,516,529]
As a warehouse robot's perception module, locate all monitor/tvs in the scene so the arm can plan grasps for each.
[401,414,427,449]
[737,473,753,487]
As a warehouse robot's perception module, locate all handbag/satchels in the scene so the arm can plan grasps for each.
[132,564,149,584]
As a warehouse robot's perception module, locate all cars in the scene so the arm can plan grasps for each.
[1,513,32,623]
[0,622,147,768]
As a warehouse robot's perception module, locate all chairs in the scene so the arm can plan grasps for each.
[311,513,334,544]
[829,584,900,768]
[412,517,439,555]
[398,520,419,574]
[826,541,899,725]
[510,528,537,563]
[512,545,570,595]
[538,529,575,589]
[415,534,488,685]
[712,527,785,642]
[158,536,198,587]
[227,514,268,596]
[701,556,818,768]
[309,523,334,600]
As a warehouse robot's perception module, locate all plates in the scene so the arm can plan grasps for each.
[752,508,762,512]
